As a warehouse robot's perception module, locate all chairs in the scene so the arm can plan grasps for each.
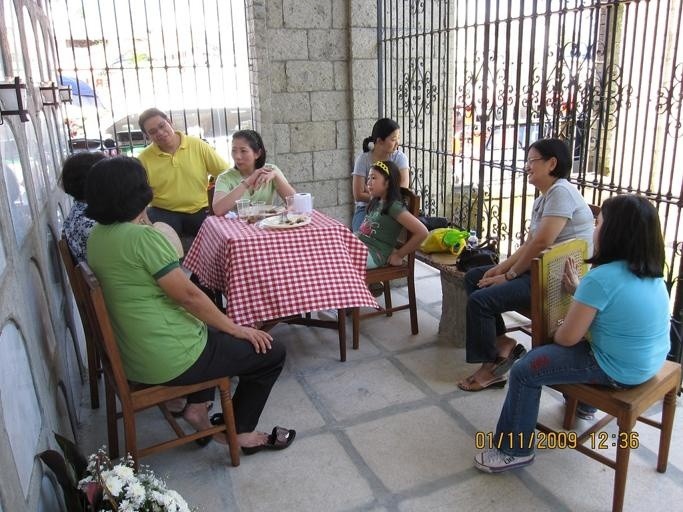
[530,238,682,511]
[77,262,240,471]
[59,240,103,410]
[345,186,419,349]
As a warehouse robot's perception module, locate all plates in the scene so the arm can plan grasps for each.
[240,206,286,216]
[261,216,312,228]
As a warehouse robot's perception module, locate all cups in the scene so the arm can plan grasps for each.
[287,196,305,223]
[236,200,250,223]
[252,201,265,228]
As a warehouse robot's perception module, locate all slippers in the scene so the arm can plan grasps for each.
[241,425,296,456]
[490,343,526,378]
[457,375,507,391]
[194,413,224,446]
[369,281,384,298]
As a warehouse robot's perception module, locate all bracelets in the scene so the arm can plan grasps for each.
[505,268,518,281]
[241,179,250,189]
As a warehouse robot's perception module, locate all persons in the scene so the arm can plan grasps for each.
[320,160,428,322]
[474,195,672,474]
[83,156,286,447]
[351,118,410,233]
[61,151,216,431]
[135,107,233,238]
[212,130,297,216]
[459,138,595,392]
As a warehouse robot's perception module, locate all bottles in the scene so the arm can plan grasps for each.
[467,231,478,249]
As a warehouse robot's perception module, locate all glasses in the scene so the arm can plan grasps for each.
[524,157,544,168]
[148,121,167,136]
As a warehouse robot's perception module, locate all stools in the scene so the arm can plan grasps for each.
[180,236,223,313]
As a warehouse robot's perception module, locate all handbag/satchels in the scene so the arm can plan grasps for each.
[456,237,500,271]
[419,216,465,231]
[419,227,470,255]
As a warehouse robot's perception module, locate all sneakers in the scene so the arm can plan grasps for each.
[317,309,338,321]
[472,448,535,473]
[566,398,598,420]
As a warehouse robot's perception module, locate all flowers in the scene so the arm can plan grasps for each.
[33,430,195,512]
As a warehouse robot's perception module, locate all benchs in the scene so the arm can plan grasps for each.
[415,249,531,349]
